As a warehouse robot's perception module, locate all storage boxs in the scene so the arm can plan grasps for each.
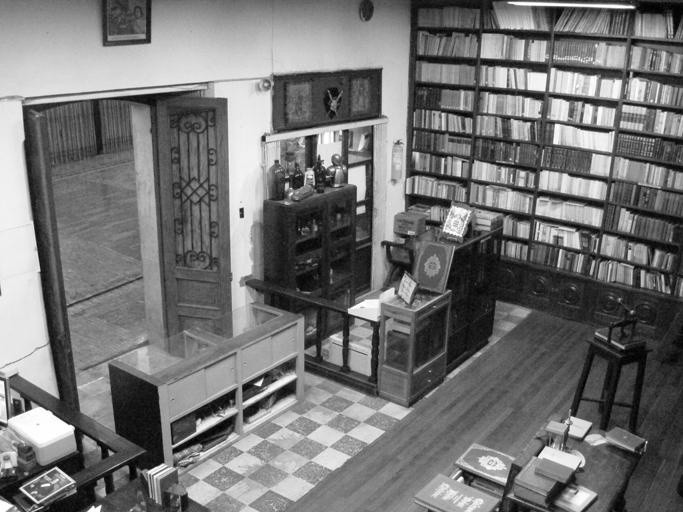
[6,407,77,466]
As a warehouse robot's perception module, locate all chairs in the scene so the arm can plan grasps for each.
[380,240,414,292]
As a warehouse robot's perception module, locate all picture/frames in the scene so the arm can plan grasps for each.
[102,0,151,46]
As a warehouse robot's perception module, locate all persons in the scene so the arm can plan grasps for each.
[110,4,146,34]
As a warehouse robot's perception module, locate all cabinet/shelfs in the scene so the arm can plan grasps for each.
[109,329,240,476]
[406,0,683,344]
[263,183,357,350]
[376,288,453,408]
[195,302,305,438]
[417,225,503,375]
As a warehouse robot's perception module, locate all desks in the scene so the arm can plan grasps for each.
[506,423,646,512]
[0,450,81,497]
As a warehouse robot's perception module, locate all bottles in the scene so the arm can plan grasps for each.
[268,160,285,200]
[293,165,303,189]
[316,172,324,193]
[303,167,315,188]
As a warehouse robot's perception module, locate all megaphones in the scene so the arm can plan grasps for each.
[258,79,275,91]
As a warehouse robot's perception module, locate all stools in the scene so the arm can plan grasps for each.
[567,336,653,435]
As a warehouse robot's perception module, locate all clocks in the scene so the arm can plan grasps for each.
[360,0,374,21]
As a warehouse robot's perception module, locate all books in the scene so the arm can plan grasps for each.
[597,12,682,296]
[405,6,481,293]
[146,463,178,507]
[606,426,646,453]
[468,1,553,261]
[564,416,593,440]
[530,7,631,278]
[414,443,598,512]
[245,408,272,424]
[12,465,77,511]
